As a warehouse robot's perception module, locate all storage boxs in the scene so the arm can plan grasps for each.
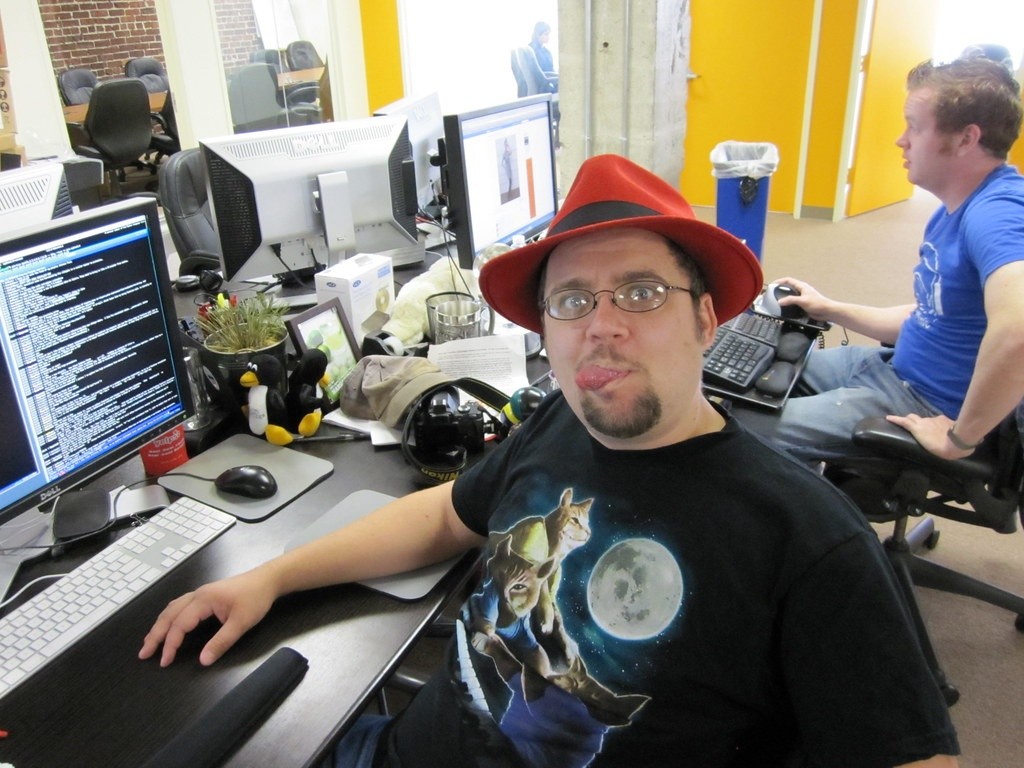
[314,252,396,352]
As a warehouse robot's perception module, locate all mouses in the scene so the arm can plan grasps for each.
[213,464,277,499]
[762,283,805,320]
[174,273,202,291]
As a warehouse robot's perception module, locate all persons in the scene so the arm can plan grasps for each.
[719,55,1024,470]
[139,155,963,768]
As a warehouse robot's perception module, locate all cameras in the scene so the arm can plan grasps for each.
[412,383,485,465]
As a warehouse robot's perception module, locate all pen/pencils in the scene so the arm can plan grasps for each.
[529,370,553,387]
[208,289,237,308]
[293,432,371,442]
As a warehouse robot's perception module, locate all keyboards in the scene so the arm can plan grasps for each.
[0,496,237,698]
[699,311,782,393]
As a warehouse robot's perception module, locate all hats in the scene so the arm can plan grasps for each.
[478,154,765,327]
[337,356,453,425]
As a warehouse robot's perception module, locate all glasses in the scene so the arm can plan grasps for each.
[538,279,698,321]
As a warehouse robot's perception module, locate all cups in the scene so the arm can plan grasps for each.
[183,345,213,432]
[427,292,495,344]
[138,423,190,477]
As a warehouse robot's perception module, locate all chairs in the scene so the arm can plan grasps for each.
[250,49,287,75]
[228,62,281,126]
[57,68,98,107]
[821,402,1024,636]
[158,147,221,277]
[510,44,559,148]
[148,87,182,174]
[124,57,169,93]
[286,41,326,72]
[285,46,345,122]
[66,78,160,208]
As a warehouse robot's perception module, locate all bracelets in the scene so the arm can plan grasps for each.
[947,424,983,449]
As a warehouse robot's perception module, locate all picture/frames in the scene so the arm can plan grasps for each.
[283,297,363,413]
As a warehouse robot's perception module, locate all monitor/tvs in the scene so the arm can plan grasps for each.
[0,94,561,602]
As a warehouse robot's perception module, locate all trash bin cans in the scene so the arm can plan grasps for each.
[709,140,780,262]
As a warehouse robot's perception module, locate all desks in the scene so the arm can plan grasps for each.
[62,66,324,127]
[0,239,828,768]
